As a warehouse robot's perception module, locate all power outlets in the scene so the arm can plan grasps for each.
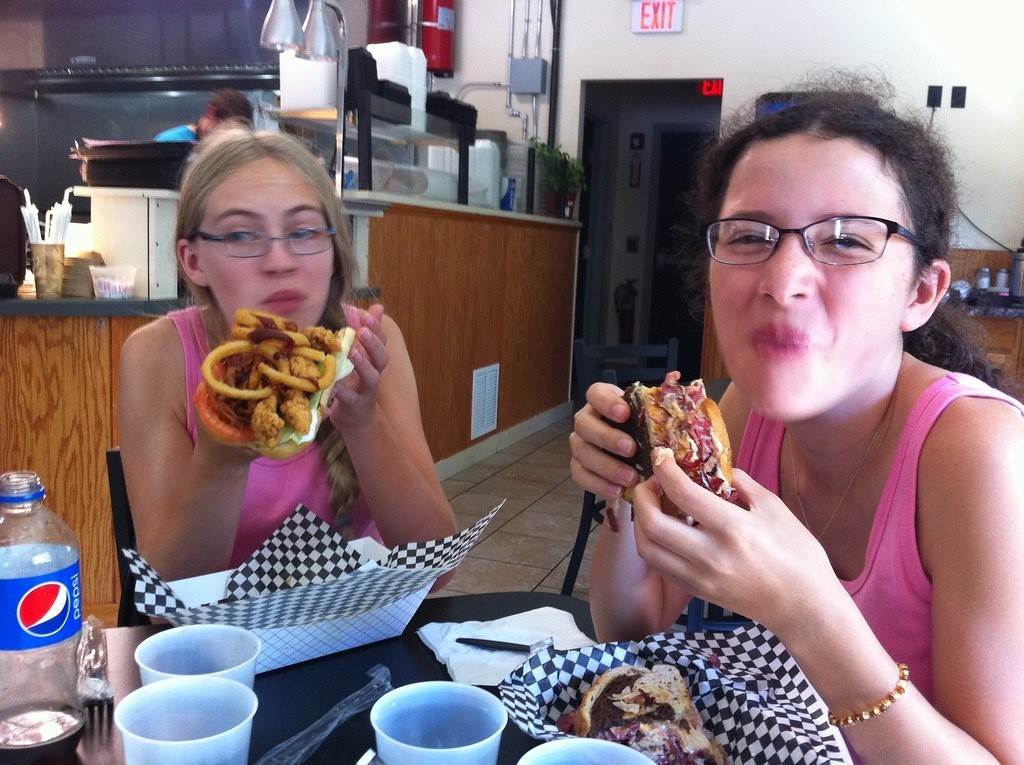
[951,87,966,108]
[927,86,942,108]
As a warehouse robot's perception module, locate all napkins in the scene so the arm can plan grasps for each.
[416,605,598,686]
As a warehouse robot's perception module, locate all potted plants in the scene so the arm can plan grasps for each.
[526,136,590,222]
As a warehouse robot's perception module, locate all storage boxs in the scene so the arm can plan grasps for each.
[122,497,512,679]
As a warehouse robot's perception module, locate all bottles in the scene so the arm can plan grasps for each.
[0,471,85,765]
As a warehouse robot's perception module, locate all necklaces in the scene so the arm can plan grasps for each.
[788,421,882,541]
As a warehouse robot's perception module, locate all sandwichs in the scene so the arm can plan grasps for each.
[574,664,732,765]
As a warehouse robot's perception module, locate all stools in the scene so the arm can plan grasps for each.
[559,335,680,599]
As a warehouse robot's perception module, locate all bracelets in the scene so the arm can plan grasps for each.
[827,660,909,729]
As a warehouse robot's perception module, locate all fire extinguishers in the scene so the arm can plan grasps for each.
[423,0,455,70]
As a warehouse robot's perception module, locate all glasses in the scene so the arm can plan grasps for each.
[704,216,924,267]
[196,222,335,257]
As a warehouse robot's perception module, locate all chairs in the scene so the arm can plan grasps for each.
[105,446,151,627]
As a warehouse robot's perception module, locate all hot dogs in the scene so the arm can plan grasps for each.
[192,308,355,460]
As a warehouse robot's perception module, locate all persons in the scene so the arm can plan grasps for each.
[117,130,458,624]
[570,90,1024,765]
[156,93,254,142]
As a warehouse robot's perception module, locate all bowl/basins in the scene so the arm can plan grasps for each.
[91,266,136,300]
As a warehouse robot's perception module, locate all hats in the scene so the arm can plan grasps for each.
[204,88,256,122]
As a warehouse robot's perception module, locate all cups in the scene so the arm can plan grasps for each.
[134,624,261,689]
[370,680,508,765]
[516,737,657,765]
[114,676,258,765]
[29,243,65,298]
[501,177,522,212]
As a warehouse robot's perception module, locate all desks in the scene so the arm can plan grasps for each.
[80,592,604,765]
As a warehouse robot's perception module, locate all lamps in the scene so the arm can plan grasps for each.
[261,1,349,208]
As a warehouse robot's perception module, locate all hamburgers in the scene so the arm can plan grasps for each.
[605,371,738,523]
